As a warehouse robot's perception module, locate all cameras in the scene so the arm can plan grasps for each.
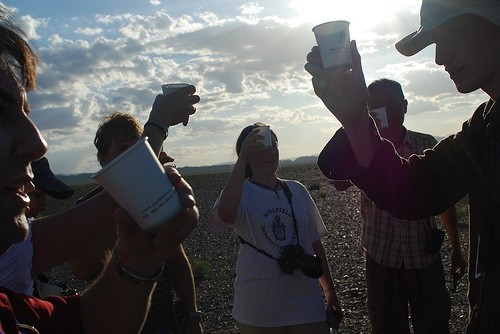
[276,245,323,279]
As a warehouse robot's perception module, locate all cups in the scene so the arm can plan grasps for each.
[161,84,189,95]
[371,107,388,128]
[252,126,272,149]
[90,136,184,232]
[312,20,353,72]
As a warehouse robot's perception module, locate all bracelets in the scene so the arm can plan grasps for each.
[324,288,335,294]
[144,120,169,140]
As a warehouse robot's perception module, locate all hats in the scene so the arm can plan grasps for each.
[394,0,500,57]
[29,157,74,200]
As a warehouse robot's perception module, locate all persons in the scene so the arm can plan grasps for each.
[305,0,500,334]
[0,23,465,334]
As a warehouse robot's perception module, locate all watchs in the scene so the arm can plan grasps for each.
[111,242,165,287]
[186,311,203,320]
